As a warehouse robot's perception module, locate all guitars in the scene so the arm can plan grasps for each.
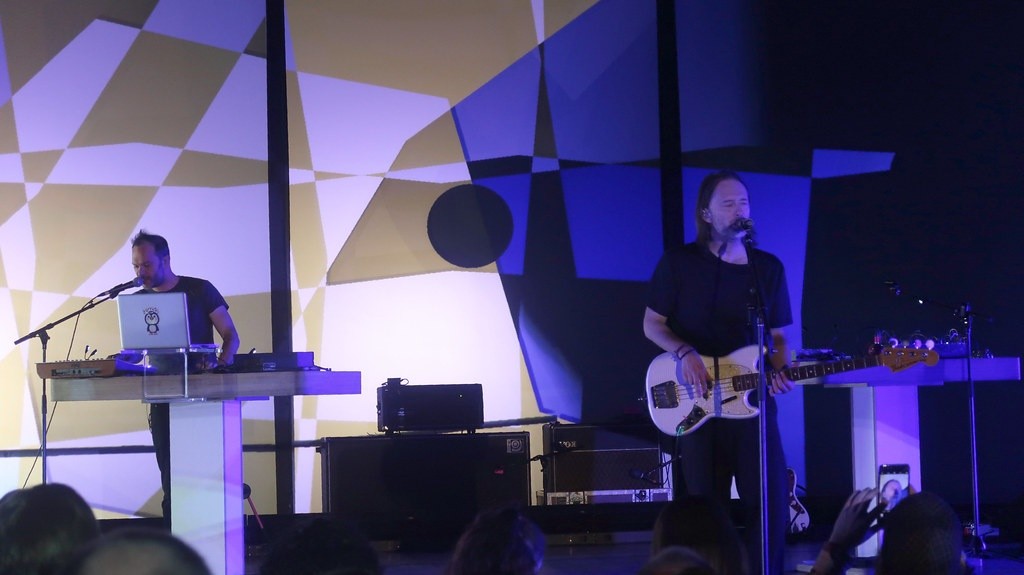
[645,338,939,437]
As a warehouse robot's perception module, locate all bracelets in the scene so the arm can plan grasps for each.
[676,344,696,360]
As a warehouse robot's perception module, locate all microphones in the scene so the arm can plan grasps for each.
[97,277,145,297]
[733,217,754,233]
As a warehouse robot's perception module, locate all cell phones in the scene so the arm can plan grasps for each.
[877,464,910,524]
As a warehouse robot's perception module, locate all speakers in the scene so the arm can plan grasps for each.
[321,432,531,523]
[541,422,664,492]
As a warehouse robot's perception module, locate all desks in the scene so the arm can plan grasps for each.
[787,355,1022,570]
[50,371,361,575]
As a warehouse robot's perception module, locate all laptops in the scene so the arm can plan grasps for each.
[118,292,219,349]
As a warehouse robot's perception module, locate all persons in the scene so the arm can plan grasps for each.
[0,483,980,575]
[643,169,801,575]
[132,232,241,520]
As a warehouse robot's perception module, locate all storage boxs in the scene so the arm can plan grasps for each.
[537,423,670,504]
[317,431,532,512]
